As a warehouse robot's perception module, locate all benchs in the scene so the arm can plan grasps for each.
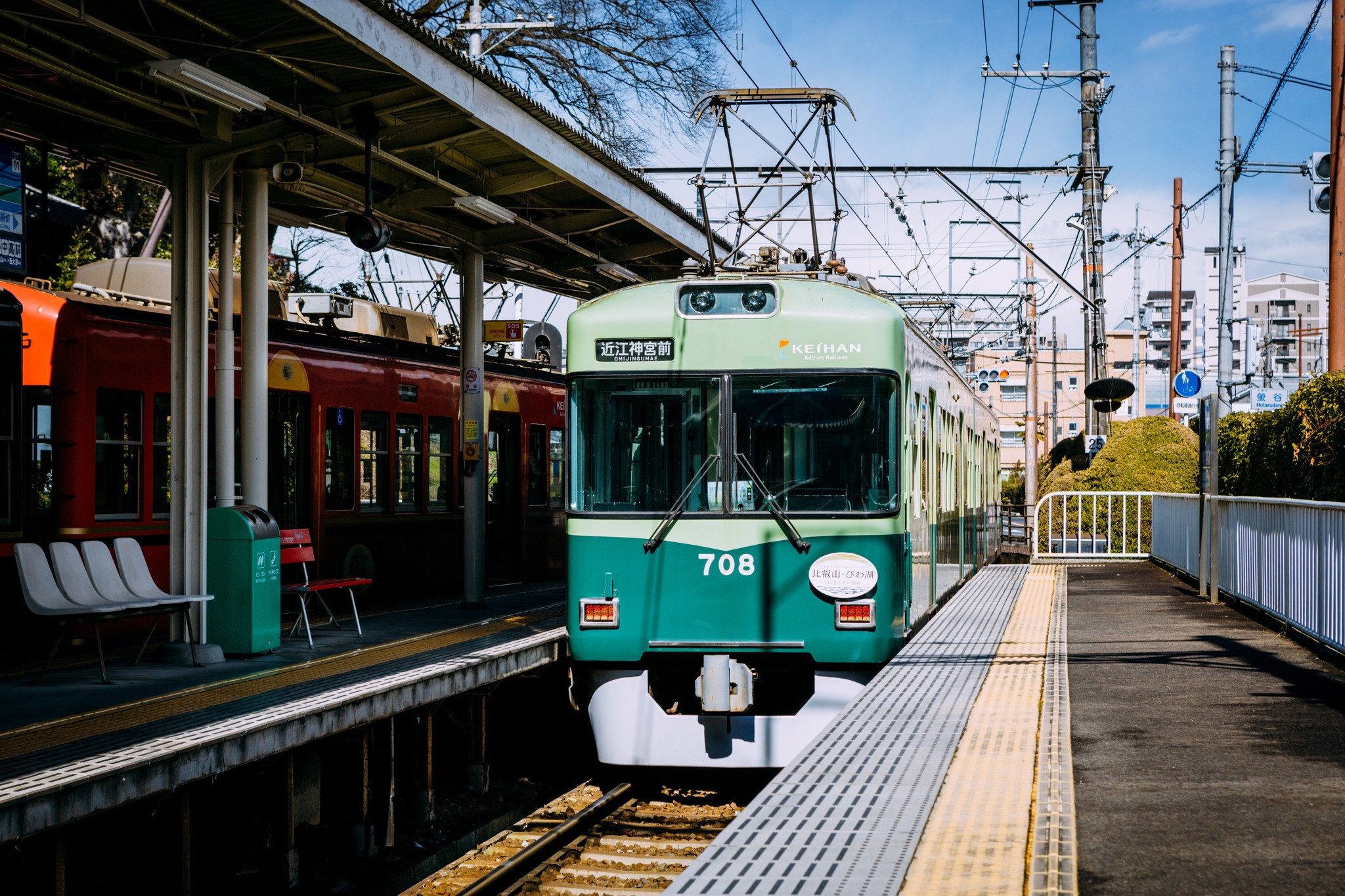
[279,524,375,651]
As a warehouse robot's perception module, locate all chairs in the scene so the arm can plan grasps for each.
[80,540,186,626]
[112,533,216,613]
[13,541,127,624]
[47,541,160,632]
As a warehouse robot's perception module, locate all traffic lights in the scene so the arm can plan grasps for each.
[977,381,989,393]
[1305,151,1331,217]
[976,369,1012,382]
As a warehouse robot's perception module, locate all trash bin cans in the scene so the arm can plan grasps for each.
[207,504,280,655]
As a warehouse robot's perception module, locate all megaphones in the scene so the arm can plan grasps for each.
[272,161,302,183]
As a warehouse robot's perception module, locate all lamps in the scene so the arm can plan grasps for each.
[139,54,272,118]
[452,194,516,228]
[593,262,639,284]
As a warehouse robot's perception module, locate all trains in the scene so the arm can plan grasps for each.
[2,256,568,656]
[560,85,1002,735]
